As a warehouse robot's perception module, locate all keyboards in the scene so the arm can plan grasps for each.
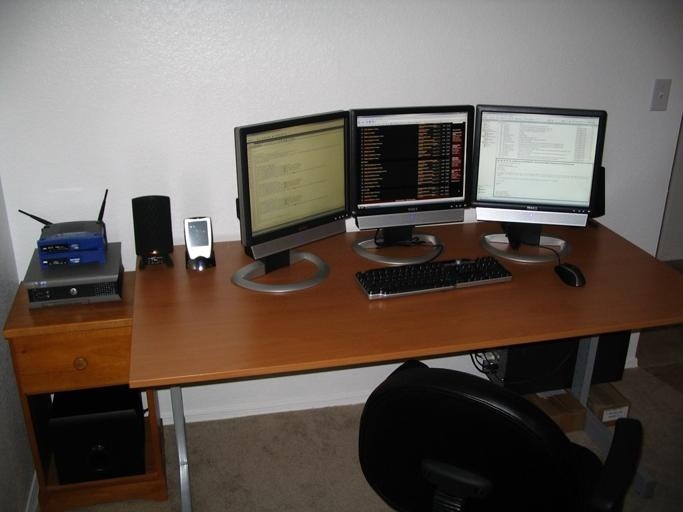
[354,255,512,299]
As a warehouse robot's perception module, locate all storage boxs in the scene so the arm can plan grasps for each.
[522,388,586,433]
[588,382,631,422]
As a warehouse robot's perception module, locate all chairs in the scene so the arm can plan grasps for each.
[359,357,643,512]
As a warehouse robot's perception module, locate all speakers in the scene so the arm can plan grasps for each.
[582,162,610,234]
[25,387,145,485]
[130,195,174,271]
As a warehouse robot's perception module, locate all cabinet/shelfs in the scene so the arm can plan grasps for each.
[3,276,168,512]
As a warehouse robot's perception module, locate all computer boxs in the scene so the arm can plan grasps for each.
[481,329,631,395]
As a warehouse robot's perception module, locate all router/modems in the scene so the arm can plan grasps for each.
[18,189,109,269]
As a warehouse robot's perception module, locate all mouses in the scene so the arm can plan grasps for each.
[553,262,586,288]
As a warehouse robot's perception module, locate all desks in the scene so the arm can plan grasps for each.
[128,214,682,512]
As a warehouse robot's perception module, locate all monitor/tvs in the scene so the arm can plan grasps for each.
[230,110,349,294]
[349,104,475,266]
[472,104,607,263]
